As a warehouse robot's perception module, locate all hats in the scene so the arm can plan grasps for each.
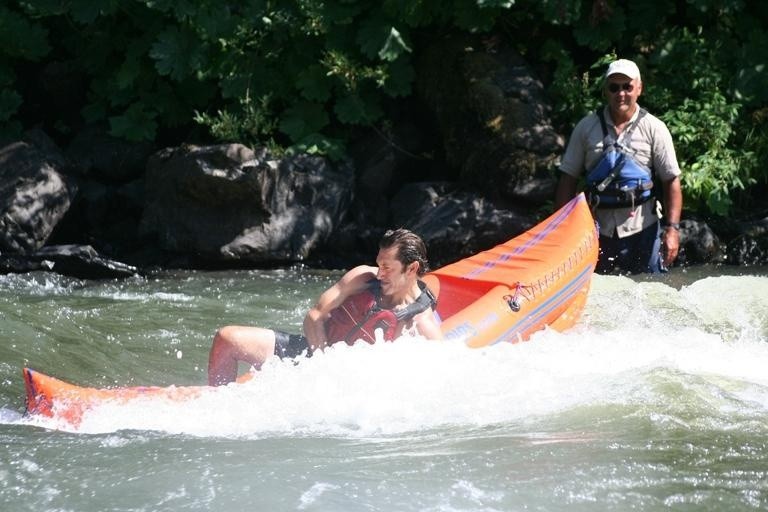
[602,56,643,83]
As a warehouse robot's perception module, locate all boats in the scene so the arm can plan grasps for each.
[24,190,599,432]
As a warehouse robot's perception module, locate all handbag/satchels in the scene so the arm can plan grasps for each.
[581,146,656,215]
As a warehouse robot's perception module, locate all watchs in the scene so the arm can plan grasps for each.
[664,223,680,230]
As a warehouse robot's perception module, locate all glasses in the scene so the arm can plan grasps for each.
[606,83,635,94]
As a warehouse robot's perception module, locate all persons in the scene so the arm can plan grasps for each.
[208,229,443,389]
[556,59,683,274]
